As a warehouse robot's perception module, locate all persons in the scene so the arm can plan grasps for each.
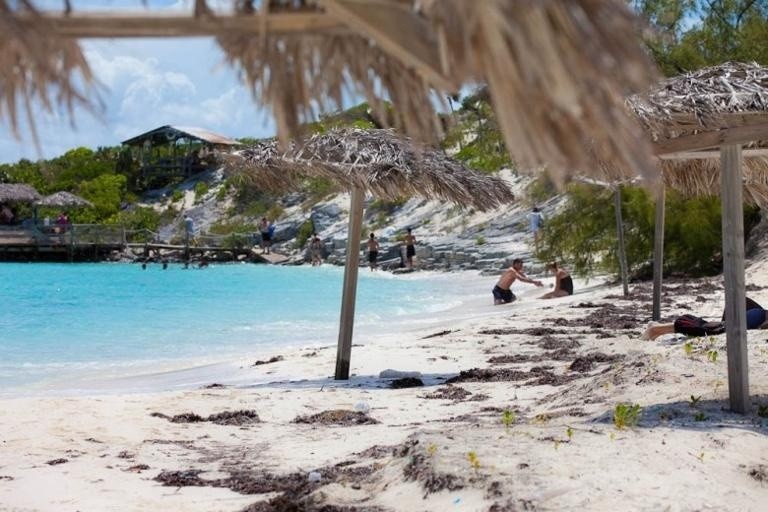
[492,259,544,305]
[183,215,198,247]
[529,207,545,255]
[538,262,573,299]
[0,203,72,242]
[641,308,768,340]
[367,233,379,272]
[310,233,321,266]
[404,228,416,268]
[259,218,271,255]
